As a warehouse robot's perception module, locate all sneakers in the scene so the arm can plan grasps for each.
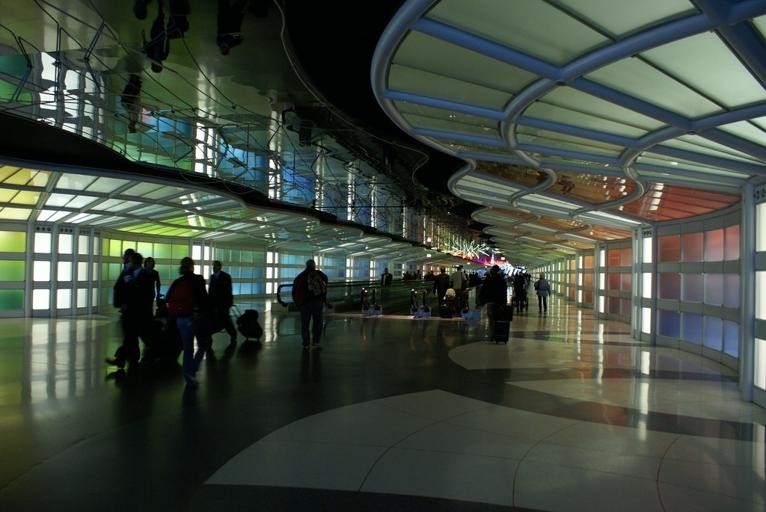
[304,342,324,351]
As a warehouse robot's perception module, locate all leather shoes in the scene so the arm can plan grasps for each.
[107,357,125,368]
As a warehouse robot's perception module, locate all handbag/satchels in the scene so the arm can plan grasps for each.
[486,303,513,320]
[463,280,471,288]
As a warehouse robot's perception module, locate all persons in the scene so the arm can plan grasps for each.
[120,64,144,136]
[132,0,246,74]
[534,272,553,314]
[291,257,329,354]
[380,263,532,332]
[103,248,238,386]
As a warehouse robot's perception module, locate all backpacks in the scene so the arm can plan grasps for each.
[446,289,455,300]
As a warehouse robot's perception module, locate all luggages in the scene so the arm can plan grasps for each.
[490,320,511,345]
[231,304,263,340]
[440,301,453,318]
